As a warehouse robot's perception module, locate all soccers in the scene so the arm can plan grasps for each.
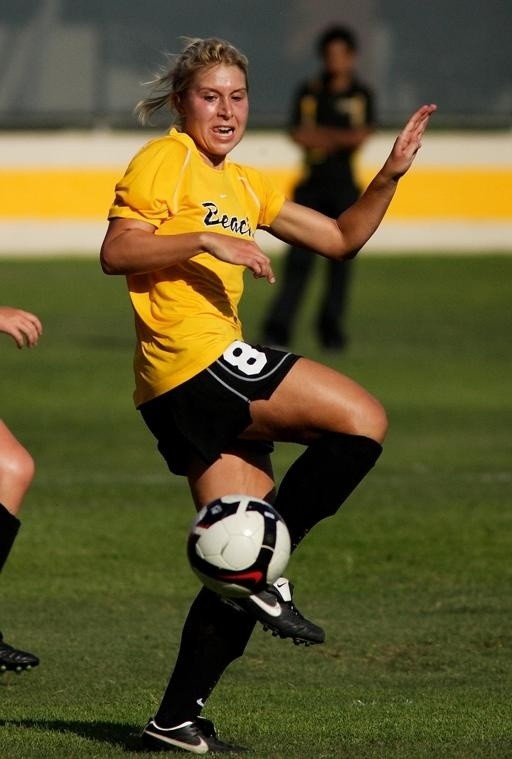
[187,495,290,599]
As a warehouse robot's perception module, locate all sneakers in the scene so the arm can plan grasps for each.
[1,641,41,674]
[138,715,249,755]
[223,577,325,647]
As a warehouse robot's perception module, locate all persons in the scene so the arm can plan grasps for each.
[101,38,437,752]
[0,307,41,672]
[260,26,379,349]
